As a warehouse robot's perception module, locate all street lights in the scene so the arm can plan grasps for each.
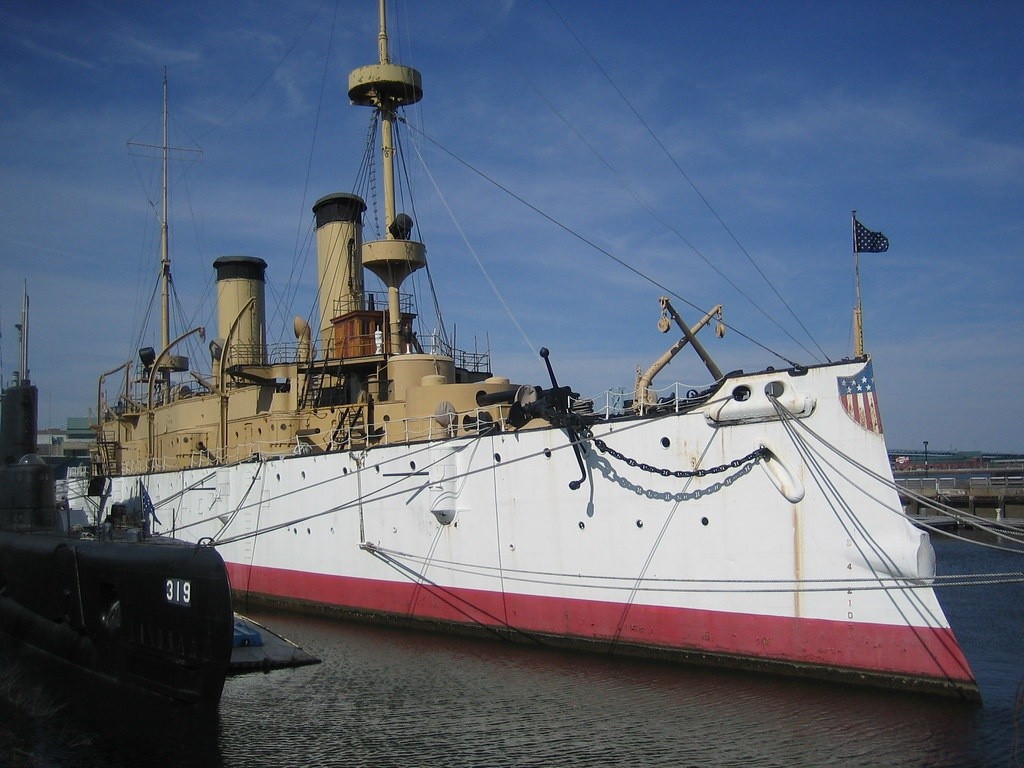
[923,441,929,476]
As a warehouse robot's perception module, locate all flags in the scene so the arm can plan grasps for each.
[140,480,162,526]
[851,216,889,255]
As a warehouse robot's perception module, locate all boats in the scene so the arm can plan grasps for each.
[46,0,977,693]
[0,272,234,709]
